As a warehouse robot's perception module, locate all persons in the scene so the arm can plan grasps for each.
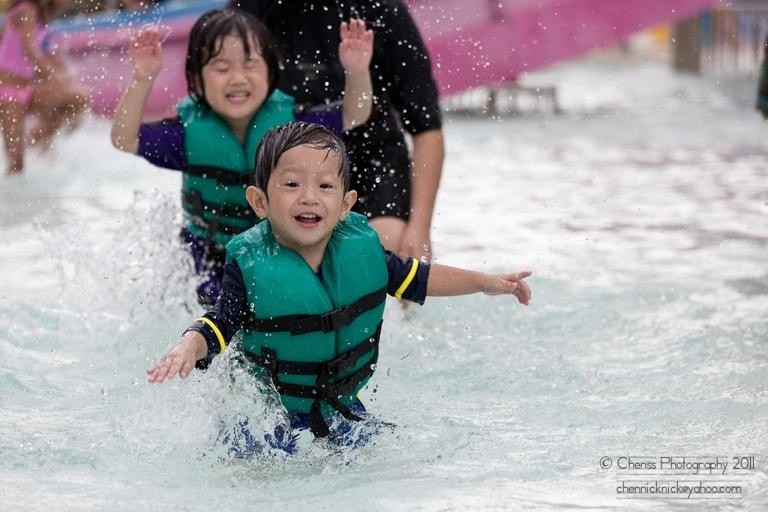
[226,1,446,314]
[143,119,533,468]
[0,1,86,174]
[107,5,385,316]
